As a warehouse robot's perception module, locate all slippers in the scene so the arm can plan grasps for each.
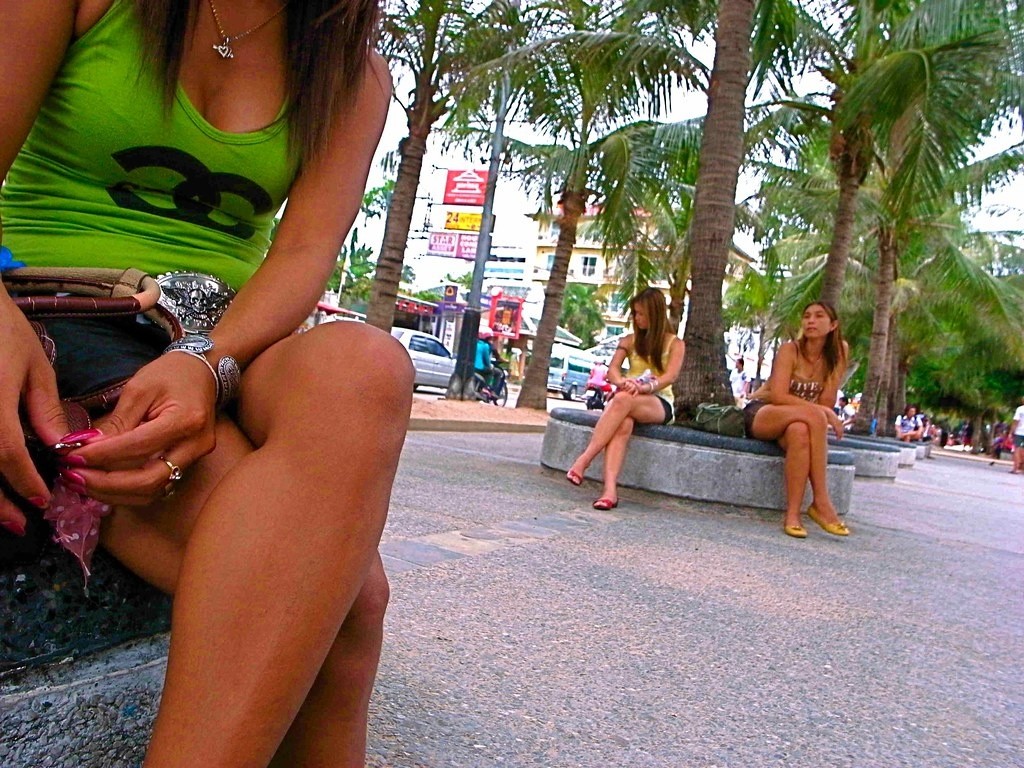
[594,498,618,510]
[566,470,584,487]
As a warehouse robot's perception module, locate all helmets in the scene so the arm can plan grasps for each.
[478,326,493,340]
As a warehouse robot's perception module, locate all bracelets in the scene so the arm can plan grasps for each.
[648,375,658,387]
[636,376,650,394]
[163,336,241,411]
[648,382,654,394]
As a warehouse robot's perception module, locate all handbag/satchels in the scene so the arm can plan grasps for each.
[690,402,746,438]
[0,264,186,444]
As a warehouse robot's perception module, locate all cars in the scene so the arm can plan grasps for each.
[391,326,458,392]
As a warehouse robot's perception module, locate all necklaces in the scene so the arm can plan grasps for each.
[211,0,286,58]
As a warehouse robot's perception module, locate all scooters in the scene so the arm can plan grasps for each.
[474,357,511,408]
[581,389,606,410]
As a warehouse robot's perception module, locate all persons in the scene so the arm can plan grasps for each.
[1,0,416,768]
[585,356,612,406]
[743,300,851,537]
[729,358,751,409]
[566,288,685,511]
[472,325,509,399]
[828,389,1024,475]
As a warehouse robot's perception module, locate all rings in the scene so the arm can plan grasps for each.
[165,482,174,496]
[159,455,185,483]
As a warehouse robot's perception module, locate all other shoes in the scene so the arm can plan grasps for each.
[807,505,850,536]
[785,526,807,538]
[491,391,499,399]
[482,388,492,398]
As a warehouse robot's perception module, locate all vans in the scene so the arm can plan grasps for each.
[549,344,600,402]
